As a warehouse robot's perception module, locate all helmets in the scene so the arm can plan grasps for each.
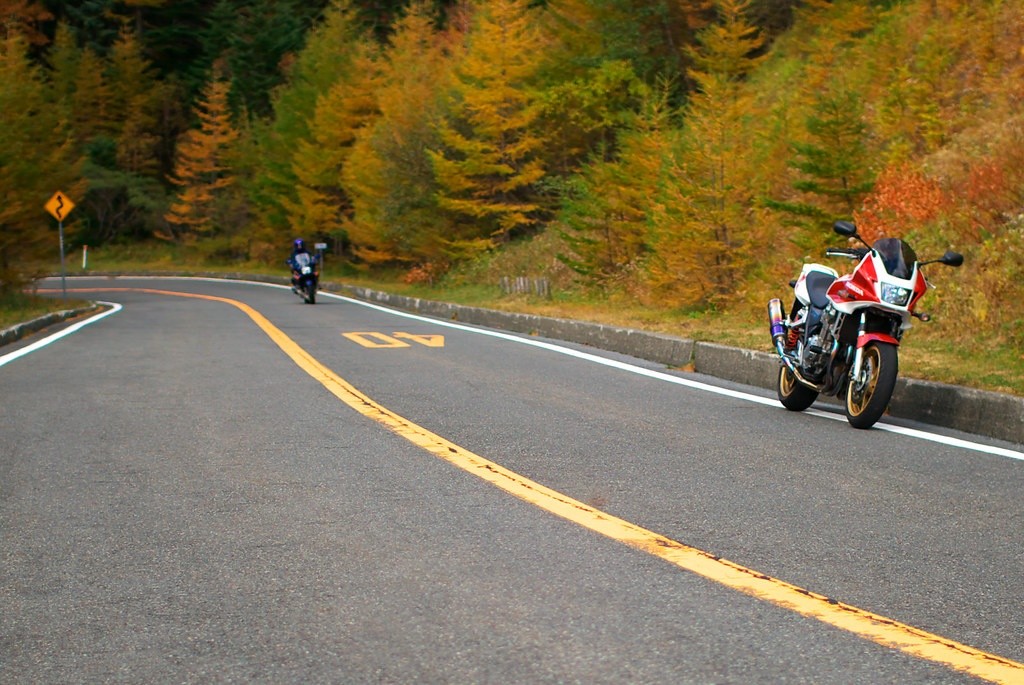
[294,239,304,252]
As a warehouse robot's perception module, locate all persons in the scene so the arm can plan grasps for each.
[287,239,312,289]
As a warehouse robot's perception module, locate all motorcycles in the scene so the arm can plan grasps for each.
[287,251,320,305]
[768,219,964,431]
